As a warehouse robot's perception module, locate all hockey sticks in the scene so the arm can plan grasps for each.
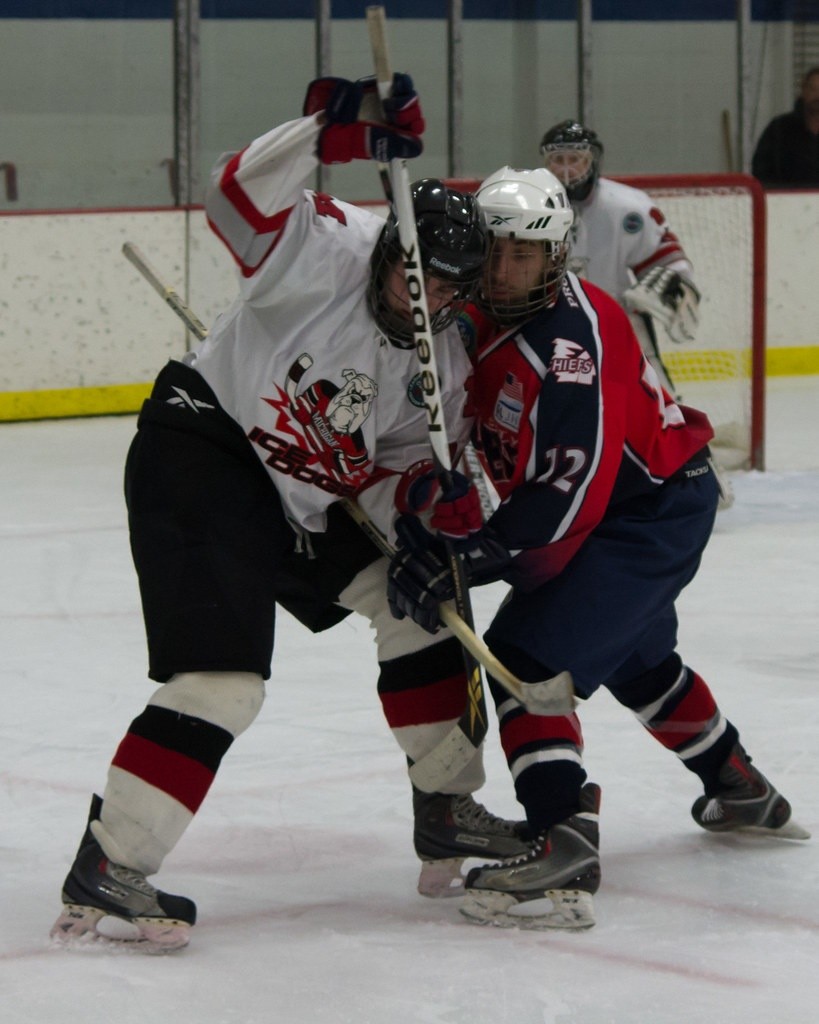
[122,237,578,719]
[360,1,488,796]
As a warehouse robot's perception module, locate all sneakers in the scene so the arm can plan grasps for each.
[42,792,196,954]
[685,738,814,849]
[406,780,542,901]
[452,783,608,944]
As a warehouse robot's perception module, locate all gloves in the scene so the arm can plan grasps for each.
[377,491,513,634]
[392,462,489,543]
[304,68,428,165]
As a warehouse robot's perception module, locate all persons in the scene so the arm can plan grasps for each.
[537,116,702,408]
[400,168,793,897]
[750,67,819,189]
[61,67,536,923]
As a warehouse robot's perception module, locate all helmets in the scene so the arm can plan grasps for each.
[386,175,496,286]
[535,118,604,160]
[465,165,582,246]
